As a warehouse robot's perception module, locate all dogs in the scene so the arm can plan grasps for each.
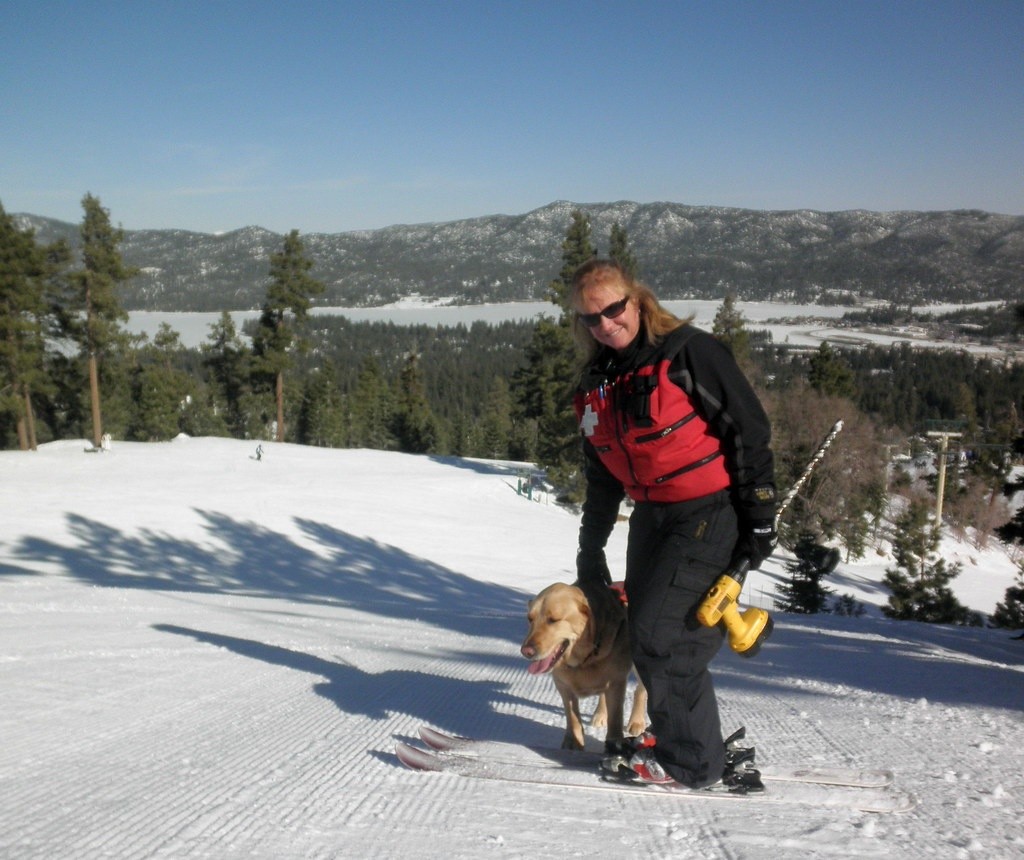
[521,580,648,750]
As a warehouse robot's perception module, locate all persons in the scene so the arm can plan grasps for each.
[567,254,778,793]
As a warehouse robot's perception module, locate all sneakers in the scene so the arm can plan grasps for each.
[592,749,723,792]
[602,729,658,756]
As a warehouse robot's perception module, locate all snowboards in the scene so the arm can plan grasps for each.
[396,727,917,813]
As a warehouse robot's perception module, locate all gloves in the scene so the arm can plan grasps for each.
[733,518,779,571]
[574,550,613,586]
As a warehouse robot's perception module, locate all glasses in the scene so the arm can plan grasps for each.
[575,294,630,329]
